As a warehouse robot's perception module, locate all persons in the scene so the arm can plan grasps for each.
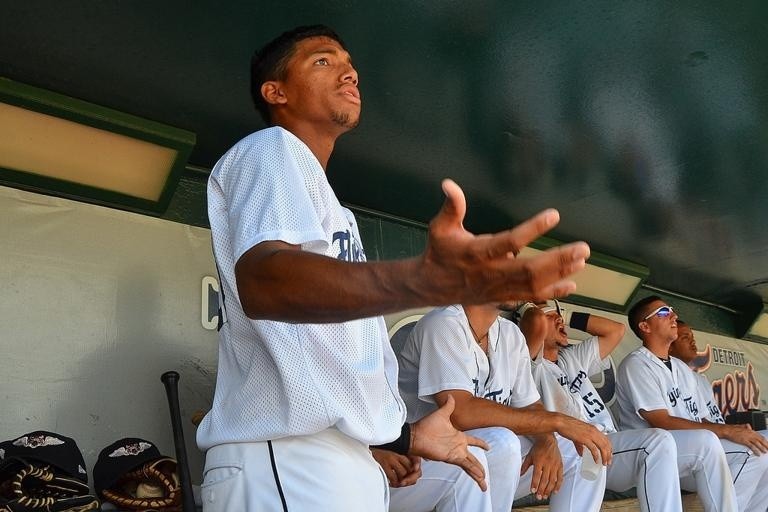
[667,313,768,437]
[193,24,591,511]
[366,435,491,510]
[497,278,741,510]
[609,292,768,512]
[396,219,616,510]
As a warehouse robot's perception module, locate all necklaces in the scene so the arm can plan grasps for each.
[469,322,487,347]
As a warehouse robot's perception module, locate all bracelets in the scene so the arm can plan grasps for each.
[568,309,590,331]
[513,299,535,321]
[369,417,413,454]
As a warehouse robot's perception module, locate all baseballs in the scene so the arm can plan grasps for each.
[136,479,162,500]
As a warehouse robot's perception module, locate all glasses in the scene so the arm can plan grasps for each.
[644,306,675,321]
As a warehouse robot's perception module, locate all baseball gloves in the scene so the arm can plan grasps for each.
[0,430,99,512]
[93,438,184,512]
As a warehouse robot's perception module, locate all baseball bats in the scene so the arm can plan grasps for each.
[161,371,198,511]
